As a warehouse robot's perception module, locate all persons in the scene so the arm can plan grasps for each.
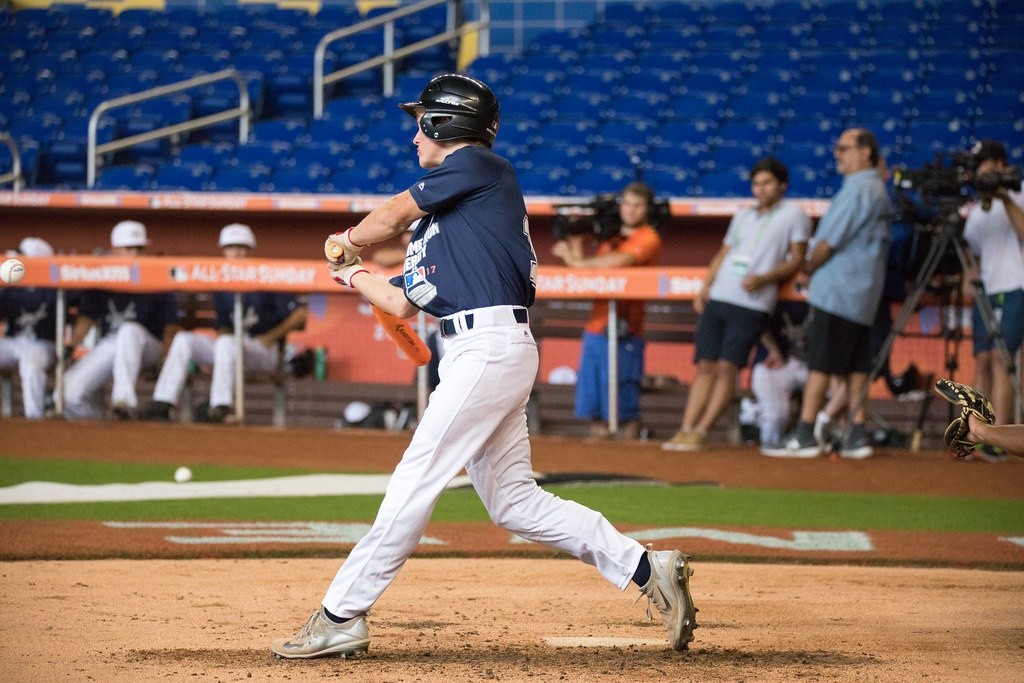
[552,181,662,447]
[134,222,307,423]
[739,125,895,458]
[667,157,811,454]
[0,235,69,419]
[51,219,170,423]
[934,375,1023,459]
[269,77,700,661]
[954,140,1023,464]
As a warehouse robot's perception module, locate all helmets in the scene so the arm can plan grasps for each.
[111,219,149,248]
[19,236,55,257]
[397,73,500,149]
[219,225,258,249]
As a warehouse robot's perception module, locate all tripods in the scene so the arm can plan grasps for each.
[832,197,1024,453]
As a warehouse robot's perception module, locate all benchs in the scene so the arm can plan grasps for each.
[1,0,1024,453]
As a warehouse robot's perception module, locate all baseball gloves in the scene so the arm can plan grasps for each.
[933,376,994,460]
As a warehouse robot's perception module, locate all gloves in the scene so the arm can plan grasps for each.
[324,227,366,270]
[329,256,370,288]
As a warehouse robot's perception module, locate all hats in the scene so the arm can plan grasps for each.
[971,141,1006,161]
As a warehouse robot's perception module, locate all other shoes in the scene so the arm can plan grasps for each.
[661,430,711,452]
[136,401,178,421]
[208,405,234,423]
[111,402,129,420]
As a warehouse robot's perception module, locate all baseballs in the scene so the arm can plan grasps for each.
[174,466,193,482]
[1,259,26,285]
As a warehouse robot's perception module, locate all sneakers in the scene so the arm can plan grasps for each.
[631,543,699,651]
[270,605,370,658]
[841,433,874,459]
[758,431,821,458]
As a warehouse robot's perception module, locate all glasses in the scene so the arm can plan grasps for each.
[836,145,853,151]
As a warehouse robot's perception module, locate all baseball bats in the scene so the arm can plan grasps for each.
[328,241,432,368]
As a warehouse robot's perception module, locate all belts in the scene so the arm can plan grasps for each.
[440,308,527,339]
